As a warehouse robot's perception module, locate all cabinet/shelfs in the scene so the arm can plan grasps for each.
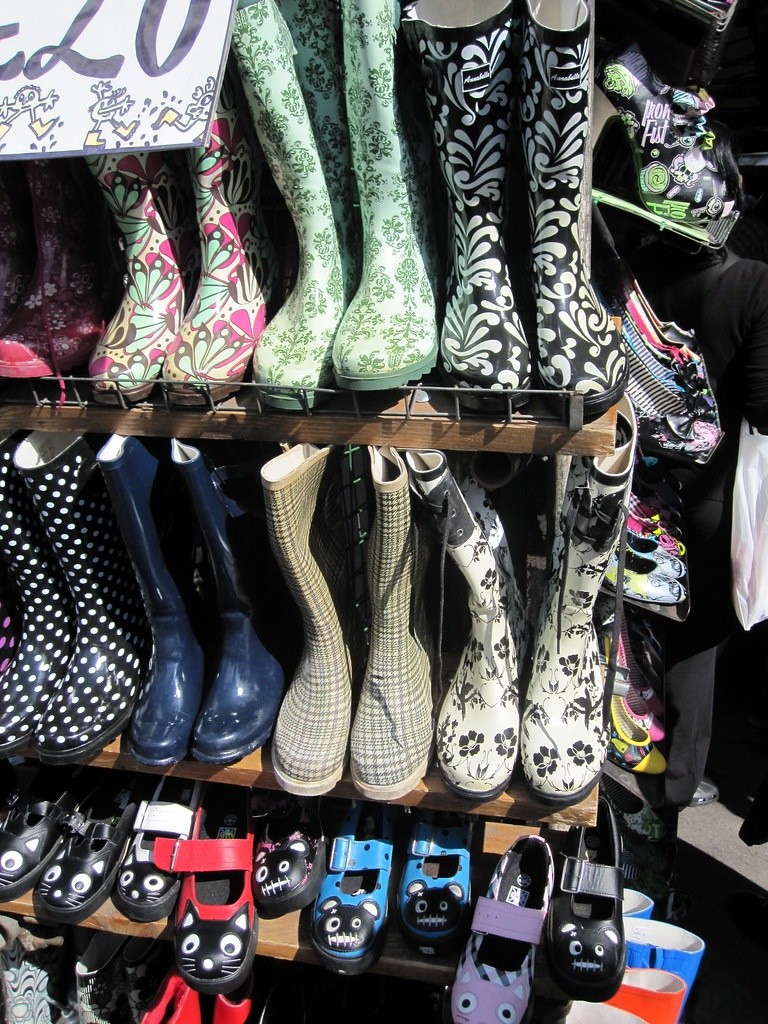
[1,303,768,1007]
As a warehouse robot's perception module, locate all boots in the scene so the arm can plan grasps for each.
[0,0,744,1024]
